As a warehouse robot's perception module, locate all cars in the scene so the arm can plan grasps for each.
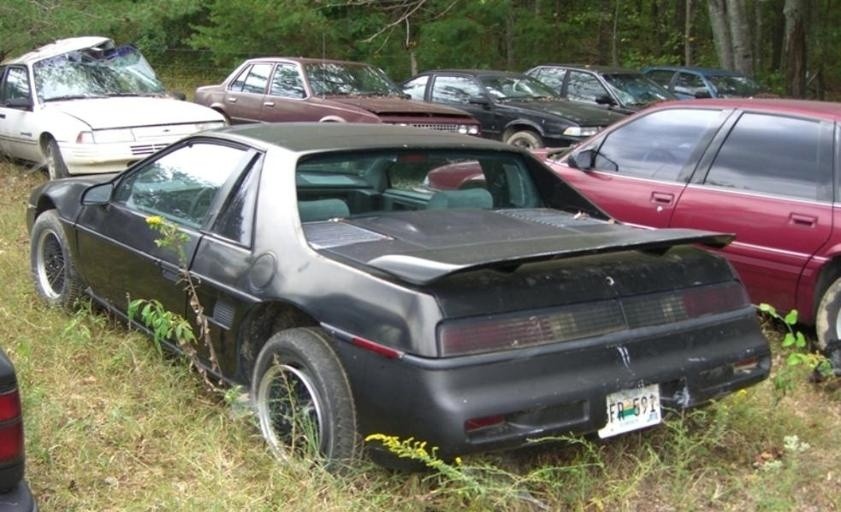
[640,64,773,102]
[1,347,39,512]
[26,122,773,491]
[386,69,630,178]
[501,64,681,115]
[0,36,230,182]
[194,57,483,176]
[421,98,841,369]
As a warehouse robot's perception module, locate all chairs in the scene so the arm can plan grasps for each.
[427,187,494,209]
[298,198,351,222]
